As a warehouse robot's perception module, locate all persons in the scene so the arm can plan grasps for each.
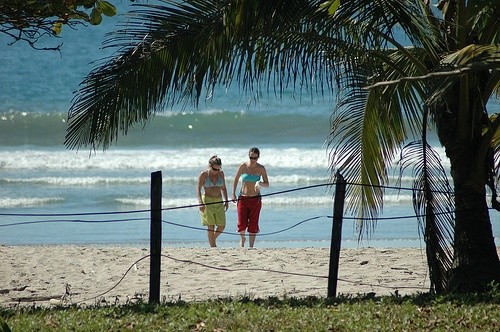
[196,156,228,248]
[231,148,270,248]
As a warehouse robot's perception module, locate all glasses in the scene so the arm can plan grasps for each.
[249,155,258,159]
[211,165,221,171]
